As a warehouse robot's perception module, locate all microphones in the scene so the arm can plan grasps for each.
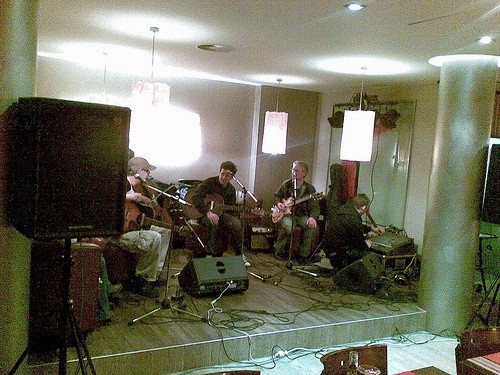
[227,170,233,175]
[292,170,297,176]
[134,174,145,183]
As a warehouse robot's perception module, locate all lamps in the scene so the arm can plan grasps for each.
[132,27,170,106]
[261,79,288,155]
[340,65,375,162]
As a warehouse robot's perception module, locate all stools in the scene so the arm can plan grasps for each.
[189,220,229,253]
[104,245,136,288]
[287,226,320,254]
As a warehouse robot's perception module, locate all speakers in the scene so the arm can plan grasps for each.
[178,256,249,297]
[0,95,131,238]
[482,144,500,225]
[331,251,385,292]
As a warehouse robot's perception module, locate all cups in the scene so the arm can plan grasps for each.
[357,364,381,375]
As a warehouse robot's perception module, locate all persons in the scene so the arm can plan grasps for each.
[58,157,172,327]
[323,193,383,266]
[274,160,318,265]
[192,161,251,267]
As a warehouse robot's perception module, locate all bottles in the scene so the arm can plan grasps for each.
[346,351,358,375]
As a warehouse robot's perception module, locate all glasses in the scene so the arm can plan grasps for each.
[143,169,150,174]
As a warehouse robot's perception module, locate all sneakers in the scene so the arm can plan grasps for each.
[236,254,251,267]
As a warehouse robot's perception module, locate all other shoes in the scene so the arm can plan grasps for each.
[296,254,308,266]
[149,278,167,286]
[108,283,123,298]
[131,279,159,298]
[96,316,119,326]
[275,252,284,259]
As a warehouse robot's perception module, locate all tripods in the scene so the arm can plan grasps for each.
[465,273,500,330]
[128,182,206,326]
[262,176,317,278]
[8,237,97,375]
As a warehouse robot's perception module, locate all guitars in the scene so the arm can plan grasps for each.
[123,199,194,238]
[271,192,324,223]
[182,188,268,220]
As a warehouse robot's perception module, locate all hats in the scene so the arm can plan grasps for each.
[128,157,156,174]
